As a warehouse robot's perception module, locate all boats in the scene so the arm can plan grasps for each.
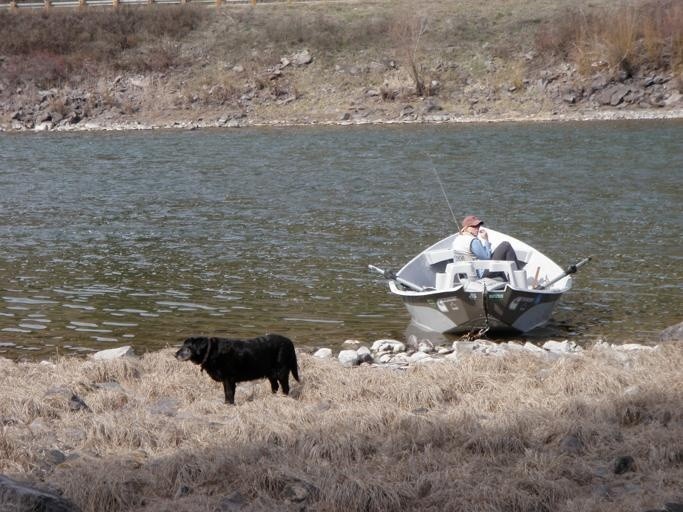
[389,225,571,338]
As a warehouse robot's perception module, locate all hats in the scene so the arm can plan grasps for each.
[462,216,483,229]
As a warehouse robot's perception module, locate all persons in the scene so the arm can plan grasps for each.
[452,216,526,281]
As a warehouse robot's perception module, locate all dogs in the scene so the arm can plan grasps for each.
[171,333,301,405]
